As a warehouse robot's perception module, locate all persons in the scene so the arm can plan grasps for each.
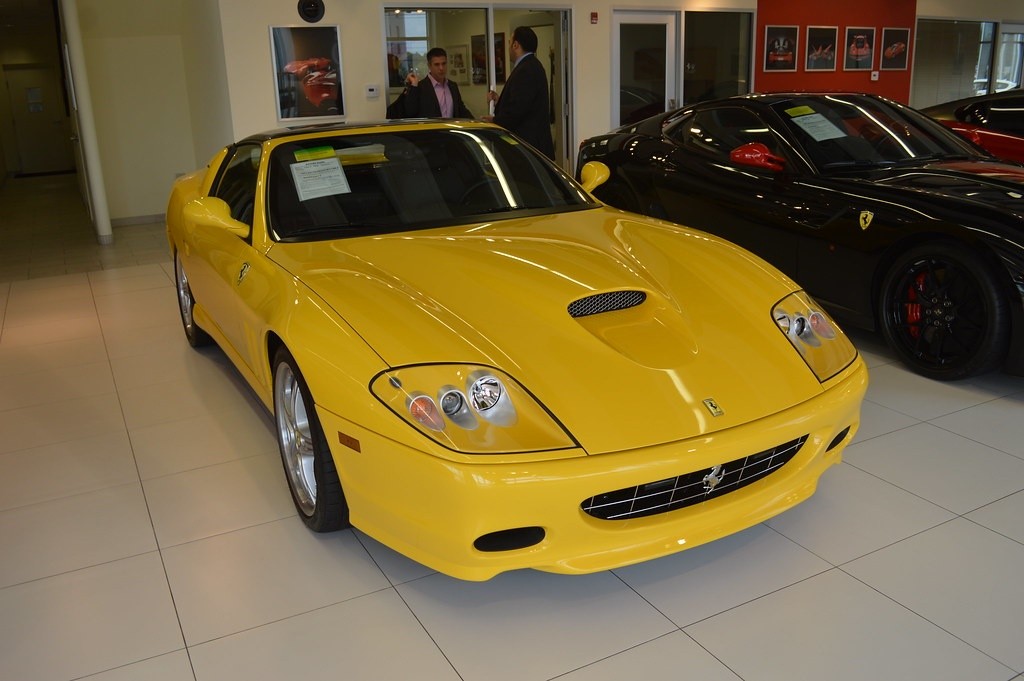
[405,48,476,119]
[387,54,405,87]
[480,27,555,162]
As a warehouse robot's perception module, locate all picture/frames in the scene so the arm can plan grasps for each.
[804,25,838,72]
[471,32,507,85]
[879,27,911,71]
[269,25,346,121]
[763,25,799,72]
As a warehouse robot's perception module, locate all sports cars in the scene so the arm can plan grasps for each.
[165,122,870,583]
[283,57,342,115]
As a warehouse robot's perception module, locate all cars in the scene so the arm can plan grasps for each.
[843,92,1024,163]
[574,91,1024,380]
[848,35,871,61]
[885,42,906,60]
